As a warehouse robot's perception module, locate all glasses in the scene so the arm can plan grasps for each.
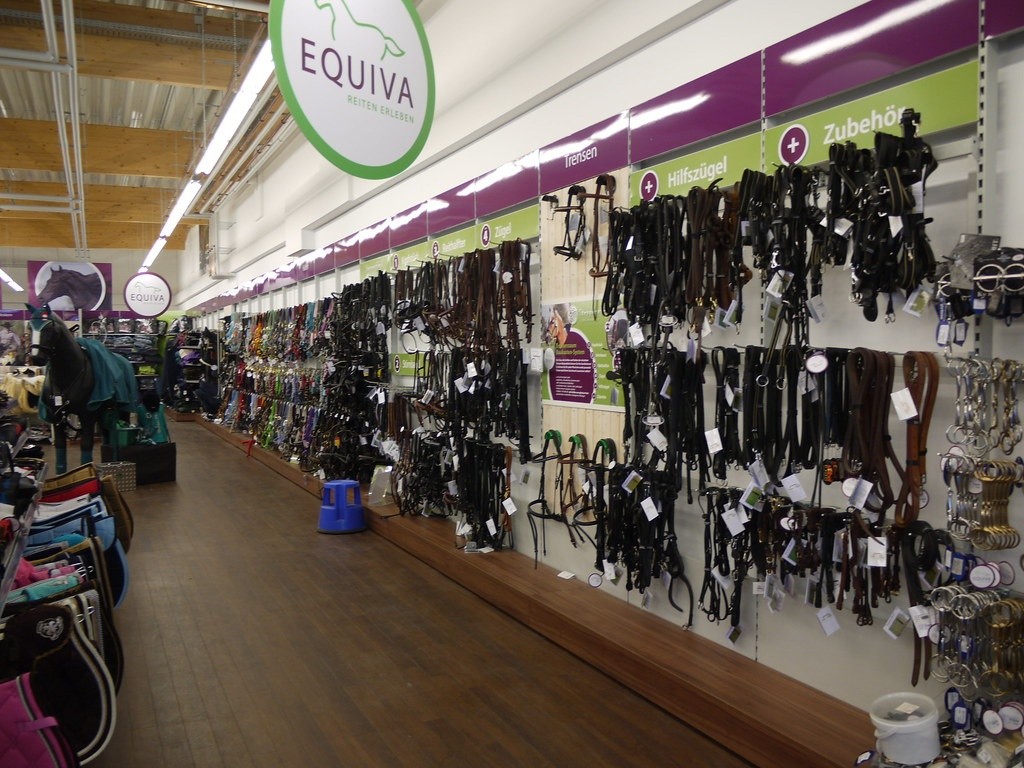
[935,248,1024,302]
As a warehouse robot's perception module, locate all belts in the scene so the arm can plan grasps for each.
[309,236,532,550]
[523,106,956,686]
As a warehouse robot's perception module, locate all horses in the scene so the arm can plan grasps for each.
[24,302,139,479]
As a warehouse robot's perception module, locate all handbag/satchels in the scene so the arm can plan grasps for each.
[118,439,178,484]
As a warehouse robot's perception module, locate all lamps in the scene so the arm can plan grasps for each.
[138,38,276,272]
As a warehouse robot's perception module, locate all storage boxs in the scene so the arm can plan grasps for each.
[95,461,136,492]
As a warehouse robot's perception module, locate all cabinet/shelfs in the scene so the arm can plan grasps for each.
[82,333,160,390]
[165,333,214,384]
[0,398,49,618]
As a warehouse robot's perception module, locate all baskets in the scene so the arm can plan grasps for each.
[93,461,136,492]
